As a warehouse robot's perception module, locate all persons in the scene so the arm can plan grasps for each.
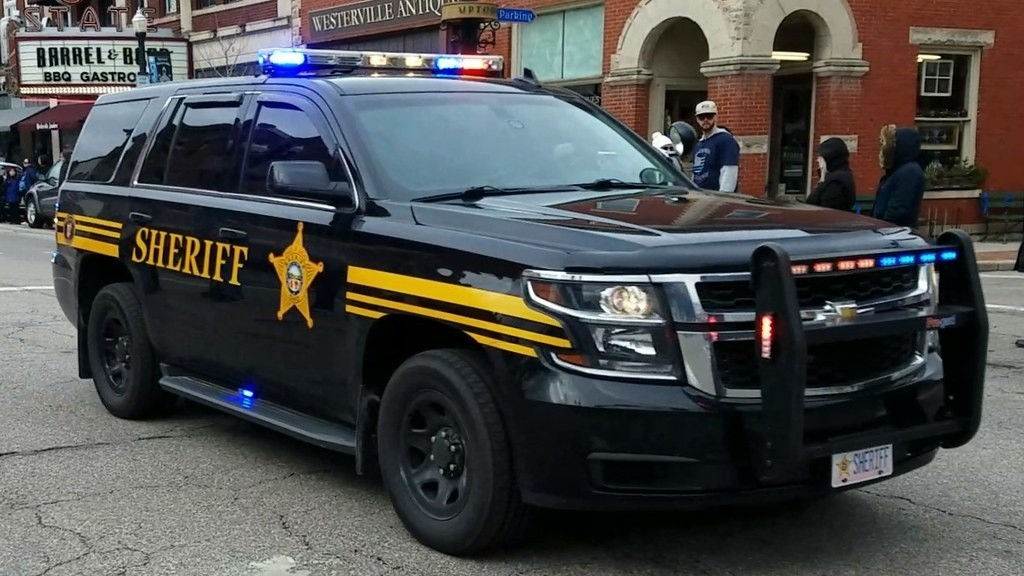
[692,101,740,192]
[871,124,926,228]
[806,137,856,212]
[0,156,51,224]
[652,132,685,201]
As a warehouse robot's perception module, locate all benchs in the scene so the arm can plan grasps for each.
[977,191,1024,244]
[851,194,927,230]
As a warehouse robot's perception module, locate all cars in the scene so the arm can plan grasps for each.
[0,157,65,230]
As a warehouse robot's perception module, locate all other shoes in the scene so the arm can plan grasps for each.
[1015,339,1024,347]
[21,222,28,225]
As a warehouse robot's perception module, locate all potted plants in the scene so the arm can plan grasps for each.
[923,156,988,188]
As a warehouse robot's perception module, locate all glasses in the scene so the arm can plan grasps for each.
[662,147,674,151]
[23,162,28,164]
[696,114,716,120]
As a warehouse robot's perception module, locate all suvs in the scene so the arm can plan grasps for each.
[51,47,988,560]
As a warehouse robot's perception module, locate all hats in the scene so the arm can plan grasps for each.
[652,132,674,150]
[695,101,718,115]
